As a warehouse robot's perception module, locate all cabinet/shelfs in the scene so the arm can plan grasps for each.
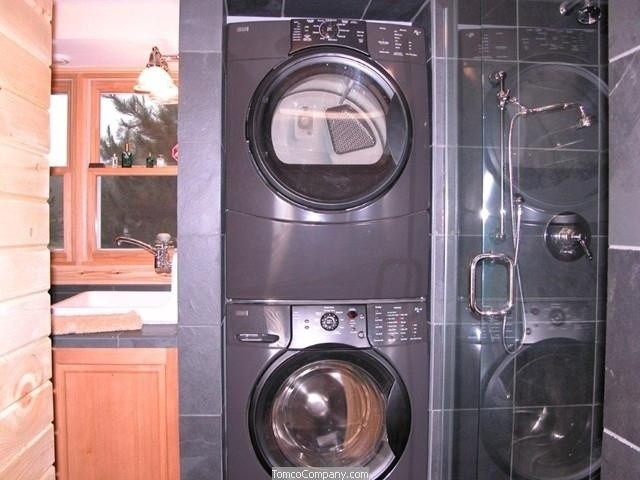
[52,348,181,480]
[50,68,178,288]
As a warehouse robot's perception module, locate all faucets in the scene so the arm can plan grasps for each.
[115,234,172,275]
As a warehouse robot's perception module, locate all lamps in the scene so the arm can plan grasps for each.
[133,45,179,99]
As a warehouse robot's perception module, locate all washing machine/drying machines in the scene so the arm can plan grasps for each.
[225,19,431,480]
[455,28,606,480]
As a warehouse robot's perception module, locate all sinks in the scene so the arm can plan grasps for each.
[51,289,179,326]
